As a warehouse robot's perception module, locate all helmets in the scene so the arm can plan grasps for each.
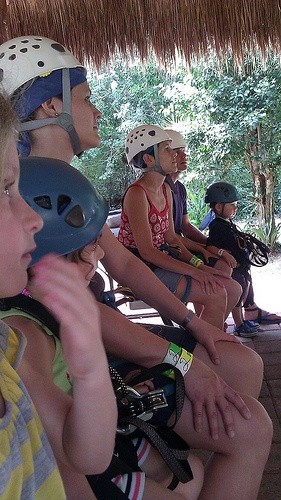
[0,36,87,101]
[164,130,186,150]
[205,182,238,203]
[17,156,108,267]
[125,125,173,164]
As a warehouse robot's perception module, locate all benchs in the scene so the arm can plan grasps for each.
[94,213,174,327]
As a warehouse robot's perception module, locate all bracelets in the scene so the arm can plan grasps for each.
[182,310,195,328]
[168,347,193,380]
[160,342,182,376]
[217,249,225,257]
[189,255,202,268]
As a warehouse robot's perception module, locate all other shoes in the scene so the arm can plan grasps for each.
[243,320,261,330]
[233,324,258,338]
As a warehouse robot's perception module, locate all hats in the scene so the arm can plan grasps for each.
[133,143,159,168]
[15,68,86,122]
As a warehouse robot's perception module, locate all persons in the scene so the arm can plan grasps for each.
[0,89,118,500]
[205,182,261,338]
[0,37,273,500]
[118,124,281,330]
[0,155,205,500]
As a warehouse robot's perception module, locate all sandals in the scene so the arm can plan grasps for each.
[245,308,281,324]
